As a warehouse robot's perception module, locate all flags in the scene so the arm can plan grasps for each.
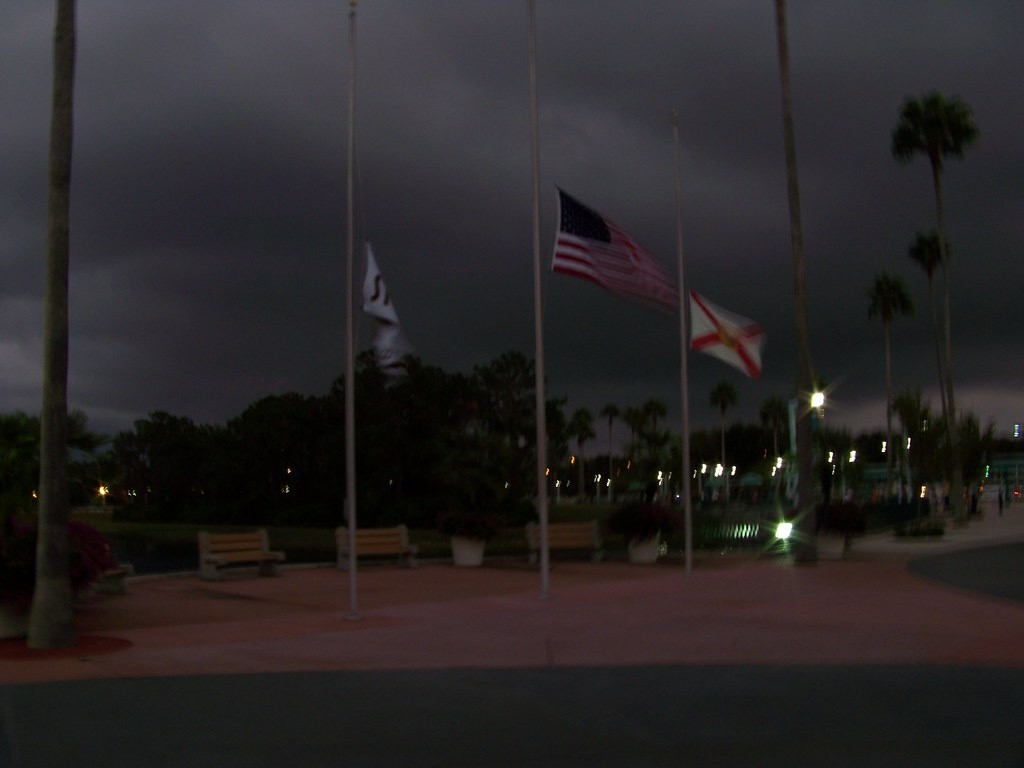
[689,290,764,378]
[362,244,411,374]
[548,183,679,305]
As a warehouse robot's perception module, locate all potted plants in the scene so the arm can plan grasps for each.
[605,500,683,565]
[439,507,501,566]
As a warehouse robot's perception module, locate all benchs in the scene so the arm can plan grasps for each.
[526,520,605,565]
[197,529,284,580]
[334,524,420,569]
